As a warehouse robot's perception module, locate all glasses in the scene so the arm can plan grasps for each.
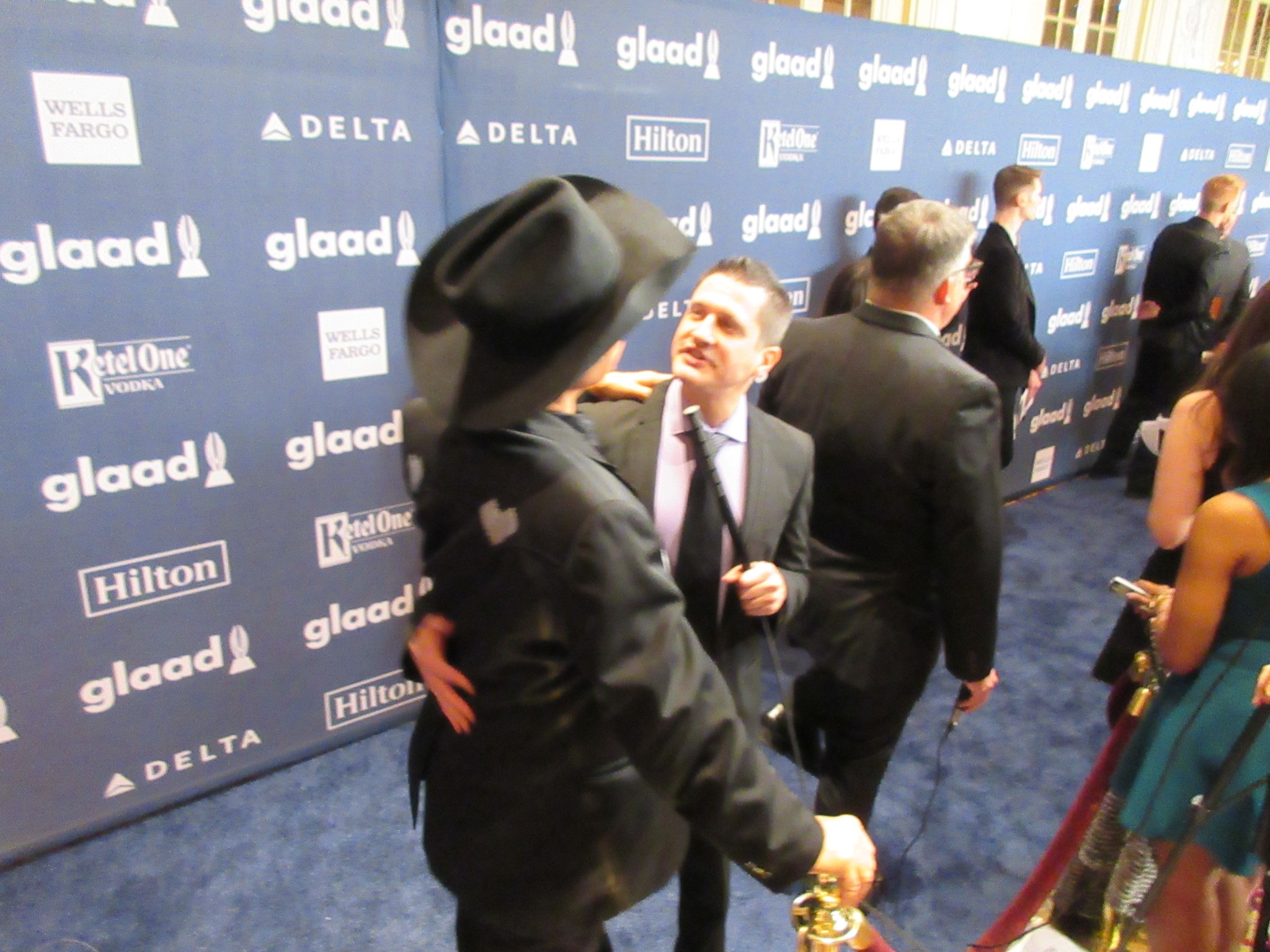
[947,258,983,278]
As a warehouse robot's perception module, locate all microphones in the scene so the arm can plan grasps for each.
[681,404,750,572]
[949,682,972,727]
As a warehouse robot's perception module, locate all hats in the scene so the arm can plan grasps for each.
[402,175,699,433]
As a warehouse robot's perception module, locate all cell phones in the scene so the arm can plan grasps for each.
[1109,576,1150,600]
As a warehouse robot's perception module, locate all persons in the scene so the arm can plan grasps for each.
[393,252,817,952]
[1087,175,1258,499]
[407,192,879,950]
[1118,343,1270,952]
[1252,662,1270,710]
[1086,282,1270,731]
[965,162,1048,471]
[825,185,919,316]
[1204,202,1255,365]
[755,198,1007,831]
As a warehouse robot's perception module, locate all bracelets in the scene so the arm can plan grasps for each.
[1147,593,1173,611]
